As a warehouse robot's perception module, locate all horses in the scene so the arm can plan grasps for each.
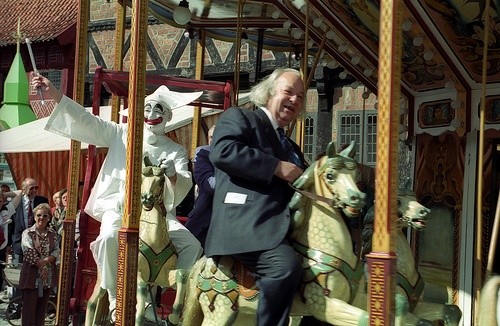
[182,141,368,326]
[358,177,462,326]
[84,156,187,326]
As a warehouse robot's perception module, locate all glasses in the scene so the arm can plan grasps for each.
[36,214,50,218]
[25,185,39,191]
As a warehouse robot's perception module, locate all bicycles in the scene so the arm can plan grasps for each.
[6,295,57,326]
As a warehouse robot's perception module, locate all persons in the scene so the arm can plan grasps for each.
[32,71,203,323]
[21,203,60,326]
[0,184,15,252]
[48,188,81,259]
[8,178,48,262]
[185,126,216,257]
[0,190,22,293]
[204,67,308,326]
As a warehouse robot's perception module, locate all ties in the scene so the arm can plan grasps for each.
[27,200,34,228]
[277,126,303,170]
